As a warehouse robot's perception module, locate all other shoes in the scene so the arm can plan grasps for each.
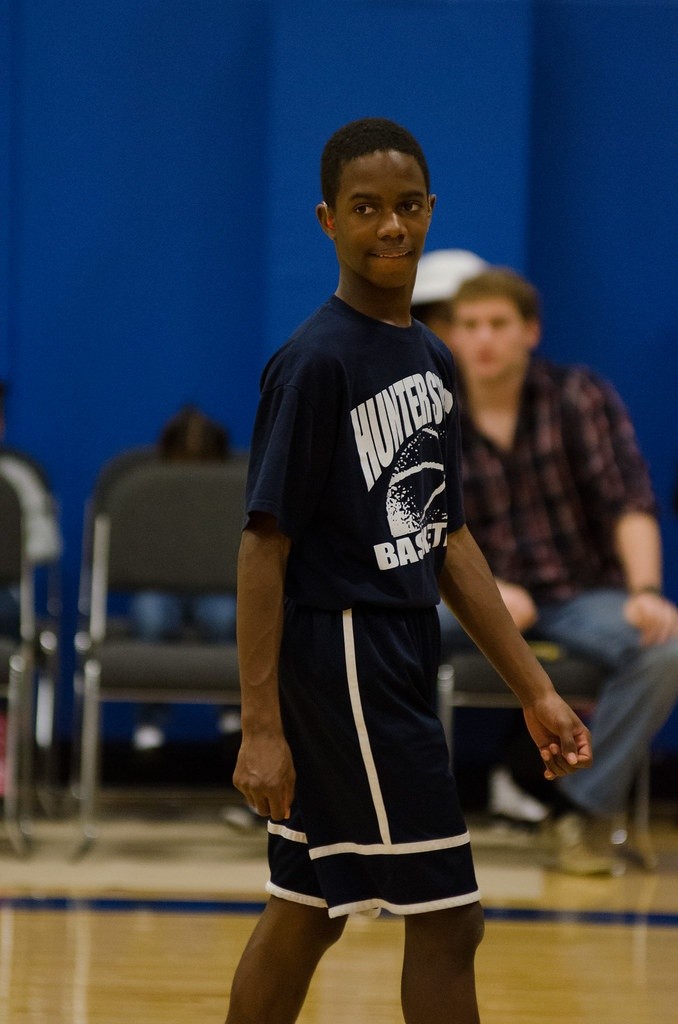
[487,770,549,822]
[536,809,615,876]
[218,800,255,832]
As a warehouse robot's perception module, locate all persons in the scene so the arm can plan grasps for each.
[401,249,678,880]
[102,404,245,750]
[223,118,593,1024]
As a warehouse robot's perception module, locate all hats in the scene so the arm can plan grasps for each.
[410,249,492,301]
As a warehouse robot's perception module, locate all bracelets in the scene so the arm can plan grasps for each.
[629,586,661,595]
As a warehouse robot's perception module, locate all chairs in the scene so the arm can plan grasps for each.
[0,464,656,876]
